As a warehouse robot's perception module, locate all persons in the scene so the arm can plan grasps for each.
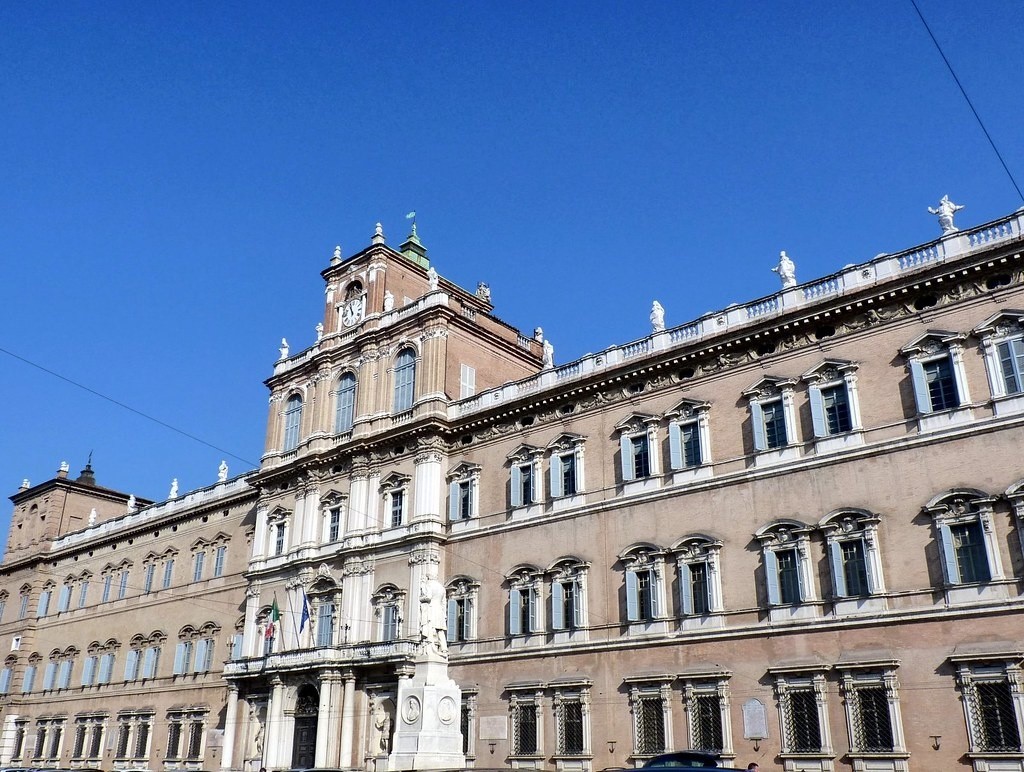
[315,323,323,340]
[927,193,964,235]
[279,338,289,359]
[418,571,448,657]
[375,712,393,754]
[427,267,438,290]
[383,290,394,311]
[771,250,796,286]
[89,508,96,525]
[476,282,491,301]
[127,494,135,513]
[650,301,665,333]
[218,460,228,481]
[168,478,178,497]
[542,340,553,368]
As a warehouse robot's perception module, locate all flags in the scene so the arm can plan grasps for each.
[406,212,415,219]
[264,598,279,640]
[299,599,309,633]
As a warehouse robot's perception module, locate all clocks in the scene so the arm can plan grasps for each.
[343,302,362,327]
